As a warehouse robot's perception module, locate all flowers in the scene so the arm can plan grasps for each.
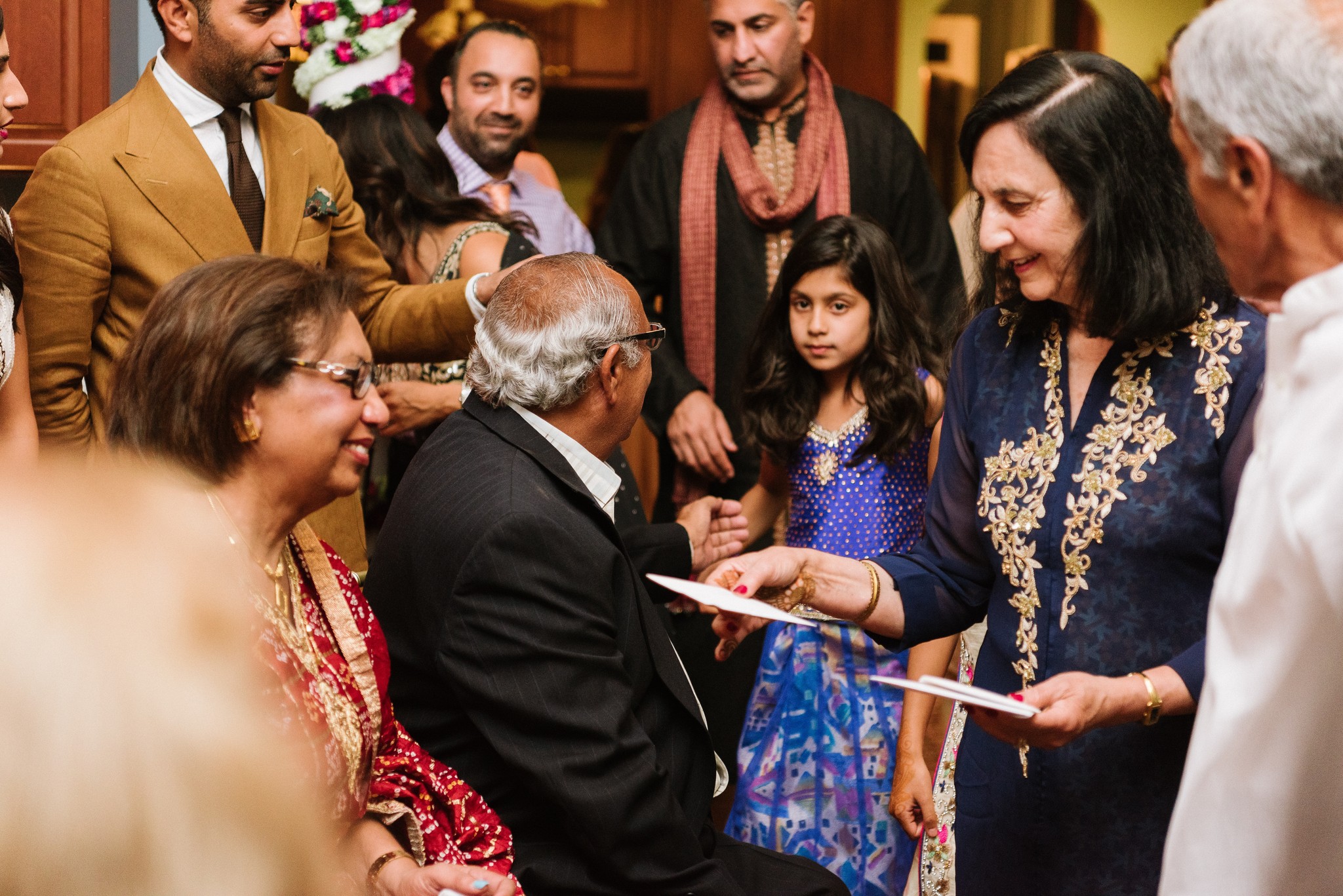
[298,0,422,110]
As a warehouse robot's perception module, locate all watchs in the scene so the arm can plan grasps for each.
[1127,671,1163,728]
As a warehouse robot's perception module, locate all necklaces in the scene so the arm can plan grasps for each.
[203,488,292,620]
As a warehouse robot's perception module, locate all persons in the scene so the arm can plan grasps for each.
[313,92,546,553]
[431,17,597,263]
[0,16,41,472]
[12,0,548,509]
[687,46,1267,895]
[90,253,531,896]
[356,249,858,896]
[1156,0,1343,896]
[658,213,951,896]
[601,0,972,541]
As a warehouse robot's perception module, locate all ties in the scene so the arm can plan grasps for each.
[216,106,266,253]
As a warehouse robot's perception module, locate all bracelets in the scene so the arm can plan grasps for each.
[365,850,415,896]
[847,559,880,627]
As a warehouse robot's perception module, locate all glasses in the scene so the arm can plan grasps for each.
[232,353,385,442]
[598,322,668,359]
